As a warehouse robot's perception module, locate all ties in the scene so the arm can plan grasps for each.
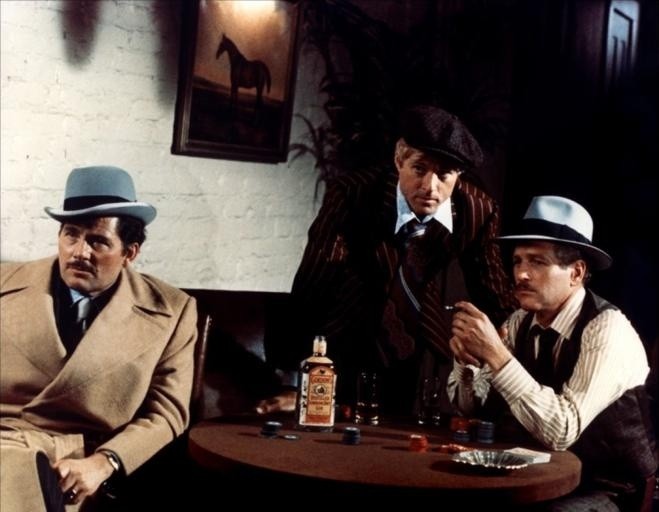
[76,297,92,331]
[537,330,559,362]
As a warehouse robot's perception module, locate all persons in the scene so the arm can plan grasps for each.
[0,165,198,512]
[446,194,659,511]
[290,106,521,431]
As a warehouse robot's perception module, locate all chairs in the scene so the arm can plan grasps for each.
[189,311,213,427]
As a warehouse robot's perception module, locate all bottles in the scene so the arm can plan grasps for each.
[295,335,337,433]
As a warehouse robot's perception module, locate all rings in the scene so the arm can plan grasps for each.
[69,491,76,500]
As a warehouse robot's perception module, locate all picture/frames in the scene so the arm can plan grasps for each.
[170,1,305,163]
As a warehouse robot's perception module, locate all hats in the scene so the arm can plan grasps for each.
[401,105,488,171]
[44,166,156,225]
[495,195,613,268]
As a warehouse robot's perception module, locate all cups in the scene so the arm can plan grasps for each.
[353,373,379,427]
[418,378,441,431]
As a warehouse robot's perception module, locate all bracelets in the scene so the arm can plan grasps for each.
[102,452,119,471]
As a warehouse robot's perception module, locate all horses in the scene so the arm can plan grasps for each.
[214,32,272,106]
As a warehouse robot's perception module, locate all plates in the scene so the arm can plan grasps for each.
[452,449,528,472]
